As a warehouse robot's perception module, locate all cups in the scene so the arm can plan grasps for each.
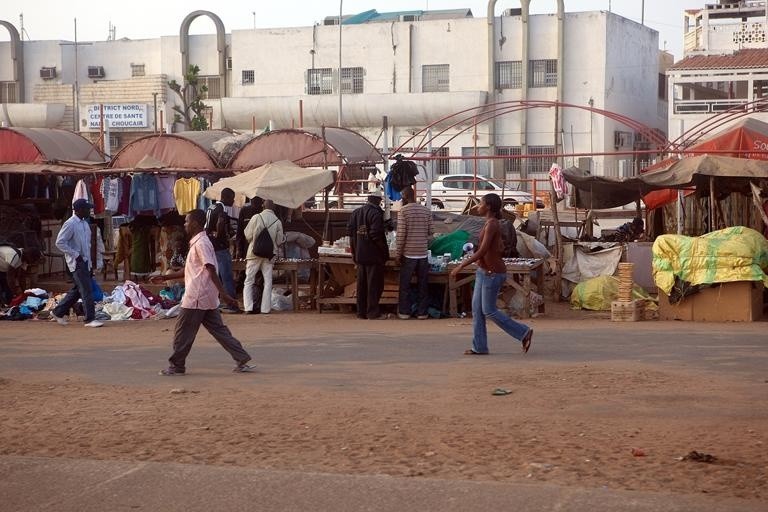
[323,241,329,248]
[334,242,339,248]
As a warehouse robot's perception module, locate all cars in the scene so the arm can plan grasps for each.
[418,174,545,212]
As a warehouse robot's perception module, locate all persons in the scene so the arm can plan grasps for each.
[159,210,257,376]
[616,219,644,242]
[347,188,395,320]
[0,245,41,316]
[243,200,284,314]
[206,188,240,313]
[396,187,435,321]
[50,199,104,328]
[166,238,187,287]
[450,193,533,355]
[237,195,263,309]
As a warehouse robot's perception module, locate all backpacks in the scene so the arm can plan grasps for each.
[253,214,279,258]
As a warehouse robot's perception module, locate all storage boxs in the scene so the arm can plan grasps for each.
[692,281,764,322]
[658,281,693,320]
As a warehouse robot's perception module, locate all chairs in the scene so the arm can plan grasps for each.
[99,249,121,280]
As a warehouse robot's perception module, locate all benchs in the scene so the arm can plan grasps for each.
[538,211,587,247]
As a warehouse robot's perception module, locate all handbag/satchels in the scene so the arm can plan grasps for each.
[0,238,17,247]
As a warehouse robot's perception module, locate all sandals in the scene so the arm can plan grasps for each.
[522,328,533,353]
[462,349,487,355]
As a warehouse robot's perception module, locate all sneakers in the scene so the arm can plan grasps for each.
[84,319,104,327]
[49,311,68,325]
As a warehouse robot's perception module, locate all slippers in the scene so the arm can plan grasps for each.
[232,364,256,374]
[158,367,185,376]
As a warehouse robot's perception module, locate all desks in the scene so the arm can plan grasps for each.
[224,256,316,311]
[449,255,546,320]
[317,249,446,318]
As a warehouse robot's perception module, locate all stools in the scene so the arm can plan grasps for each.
[44,253,64,279]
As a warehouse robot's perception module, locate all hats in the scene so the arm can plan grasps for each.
[71,198,96,210]
[367,188,381,197]
